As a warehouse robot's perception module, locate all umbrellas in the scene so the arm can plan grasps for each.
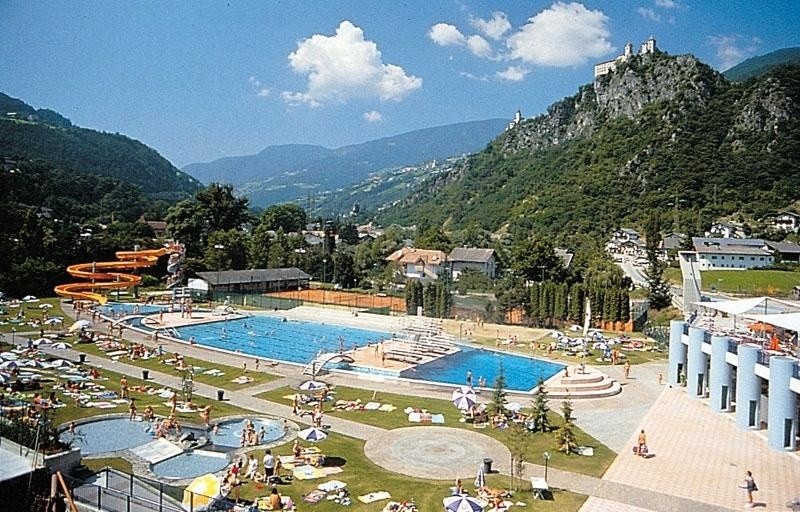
[552,324,603,348]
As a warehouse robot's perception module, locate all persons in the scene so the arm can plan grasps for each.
[624,360,631,379]
[637,429,648,458]
[742,470,758,509]
[611,348,620,365]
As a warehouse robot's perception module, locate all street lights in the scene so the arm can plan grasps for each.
[311,360,317,380]
[11,327,17,347]
[323,259,327,283]
[542,451,552,482]
[215,245,224,303]
[295,248,306,287]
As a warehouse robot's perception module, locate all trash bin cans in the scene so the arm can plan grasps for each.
[33,345,37,349]
[483,458,493,473]
[143,371,148,379]
[79,354,86,362]
[217,390,224,401]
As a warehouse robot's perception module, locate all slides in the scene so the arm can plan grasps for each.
[53,248,167,304]
